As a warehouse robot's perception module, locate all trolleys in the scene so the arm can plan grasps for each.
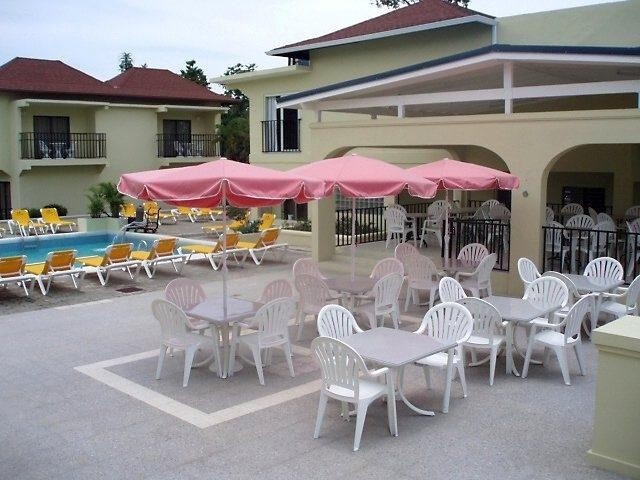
[126,207,162,234]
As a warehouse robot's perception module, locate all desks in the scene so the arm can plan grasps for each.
[183,142,194,156]
[188,295,260,380]
[50,142,67,158]
[336,325,459,438]
[480,295,563,374]
[326,273,376,318]
[563,274,626,337]
[434,263,474,282]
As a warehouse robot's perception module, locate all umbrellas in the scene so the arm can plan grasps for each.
[116,157,337,358]
[405,158,520,263]
[284,153,439,307]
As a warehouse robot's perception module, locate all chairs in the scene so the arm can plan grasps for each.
[517,257,541,291]
[117,199,276,236]
[369,258,405,325]
[401,301,475,414]
[163,277,209,358]
[173,140,185,156]
[252,278,292,310]
[455,253,498,299]
[292,257,328,286]
[230,296,297,387]
[590,275,640,341]
[39,207,76,233]
[76,242,142,286]
[310,336,398,452]
[584,257,624,280]
[0,254,33,297]
[365,272,405,330]
[27,249,86,296]
[237,228,288,266]
[194,140,204,157]
[131,237,189,279]
[8,208,45,236]
[316,304,365,422]
[294,272,332,344]
[524,276,568,333]
[394,243,420,259]
[541,271,580,304]
[523,295,590,387]
[180,232,242,271]
[404,255,438,312]
[150,297,223,387]
[457,243,489,261]
[439,275,467,303]
[454,297,519,389]
[38,139,52,158]
[65,140,77,158]
[382,197,640,272]
